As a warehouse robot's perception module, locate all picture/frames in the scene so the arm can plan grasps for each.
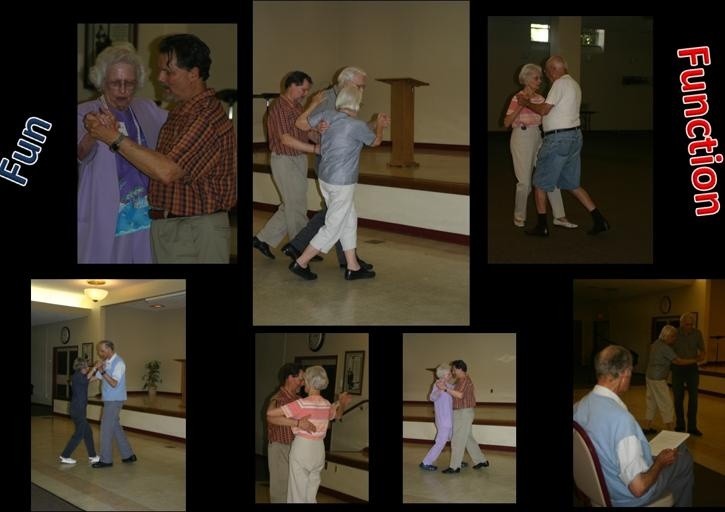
[85,24,137,89]
[82,343,94,367]
[343,351,364,395]
[690,312,698,329]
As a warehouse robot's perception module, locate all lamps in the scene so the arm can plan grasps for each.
[84,287,109,302]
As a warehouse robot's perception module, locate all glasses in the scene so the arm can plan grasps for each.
[103,79,137,91]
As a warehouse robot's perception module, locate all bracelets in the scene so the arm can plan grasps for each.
[444,387,448,391]
[297,419,300,426]
[94,367,98,371]
[101,371,106,375]
[526,102,530,107]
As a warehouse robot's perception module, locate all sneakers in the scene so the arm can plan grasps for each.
[88,456,101,463]
[59,456,76,464]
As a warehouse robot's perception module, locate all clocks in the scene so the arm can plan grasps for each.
[660,297,671,313]
[306,333,325,351]
[61,327,70,344]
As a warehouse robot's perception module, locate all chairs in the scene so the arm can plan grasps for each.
[573,421,674,507]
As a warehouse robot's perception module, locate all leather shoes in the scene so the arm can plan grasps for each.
[420,463,438,472]
[452,407,461,410]
[280,244,325,262]
[343,267,376,282]
[521,226,549,237]
[122,454,137,463]
[472,460,489,470]
[687,427,702,438]
[586,220,610,236]
[442,468,460,474]
[552,217,579,229]
[253,236,276,260]
[288,260,318,280]
[513,218,526,228]
[461,462,468,468]
[338,259,373,273]
[642,428,657,437]
[91,461,113,469]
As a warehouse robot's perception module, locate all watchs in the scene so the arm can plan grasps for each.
[109,133,125,153]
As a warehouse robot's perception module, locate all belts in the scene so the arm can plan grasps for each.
[148,209,228,219]
[542,125,581,137]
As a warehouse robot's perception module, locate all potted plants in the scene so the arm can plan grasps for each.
[143,359,162,399]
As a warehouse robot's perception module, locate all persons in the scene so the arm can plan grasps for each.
[436,360,489,473]
[58,358,104,464]
[82,33,238,264]
[419,363,468,471]
[516,55,611,236]
[77,40,170,264]
[504,63,580,229]
[573,311,705,507]
[68,340,138,468]
[253,34,392,281]
